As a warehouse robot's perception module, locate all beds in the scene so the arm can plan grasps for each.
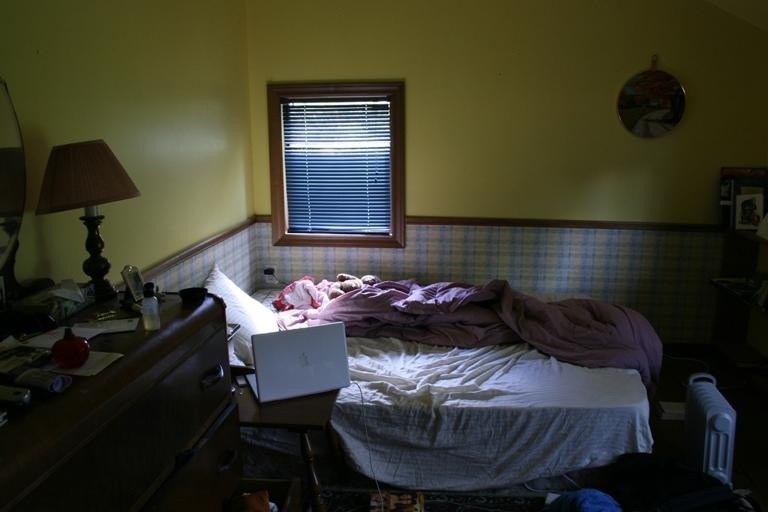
[239,286,654,491]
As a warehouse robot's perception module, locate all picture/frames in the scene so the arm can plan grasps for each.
[120,264,146,302]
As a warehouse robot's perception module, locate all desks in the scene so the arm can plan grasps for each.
[232,373,341,506]
[0,291,242,512]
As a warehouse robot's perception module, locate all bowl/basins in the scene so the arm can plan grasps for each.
[178,286,207,305]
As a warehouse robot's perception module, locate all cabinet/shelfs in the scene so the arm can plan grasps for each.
[707,229,768,370]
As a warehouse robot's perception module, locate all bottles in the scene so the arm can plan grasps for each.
[142,282,161,332]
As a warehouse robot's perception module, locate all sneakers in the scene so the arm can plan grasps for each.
[524,478,567,493]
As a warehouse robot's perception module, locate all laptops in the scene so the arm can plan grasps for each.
[245,321,350,405]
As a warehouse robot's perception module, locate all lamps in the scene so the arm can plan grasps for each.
[0,147,25,236]
[34,139,140,302]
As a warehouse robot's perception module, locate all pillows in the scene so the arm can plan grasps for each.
[202,263,279,368]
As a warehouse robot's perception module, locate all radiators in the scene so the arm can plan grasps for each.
[685,373,738,490]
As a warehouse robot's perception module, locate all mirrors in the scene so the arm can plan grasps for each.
[617,53,687,139]
[0,74,57,308]
